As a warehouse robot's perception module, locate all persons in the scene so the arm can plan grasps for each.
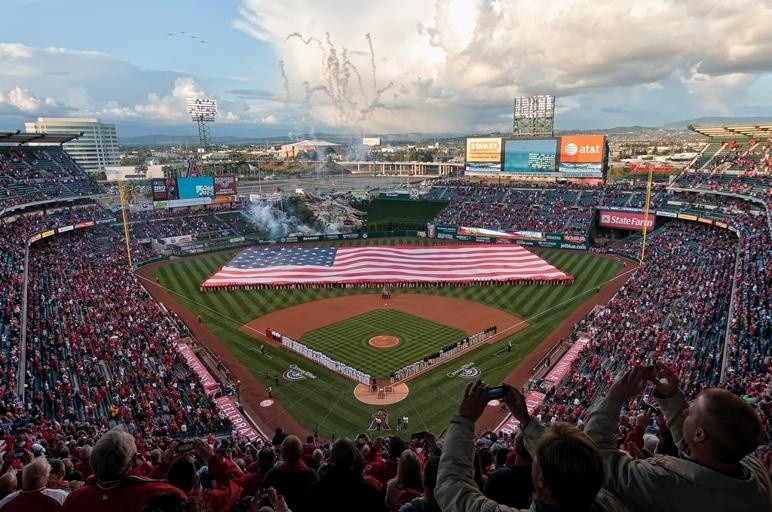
[0,136,772,511]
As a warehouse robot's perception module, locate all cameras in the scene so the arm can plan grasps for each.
[637,399,661,417]
[482,385,506,400]
[643,366,658,379]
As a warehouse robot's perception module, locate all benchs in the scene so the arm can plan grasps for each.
[1,142,233,477]
[411,133,770,474]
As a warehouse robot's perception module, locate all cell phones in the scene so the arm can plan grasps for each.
[176,441,195,453]
